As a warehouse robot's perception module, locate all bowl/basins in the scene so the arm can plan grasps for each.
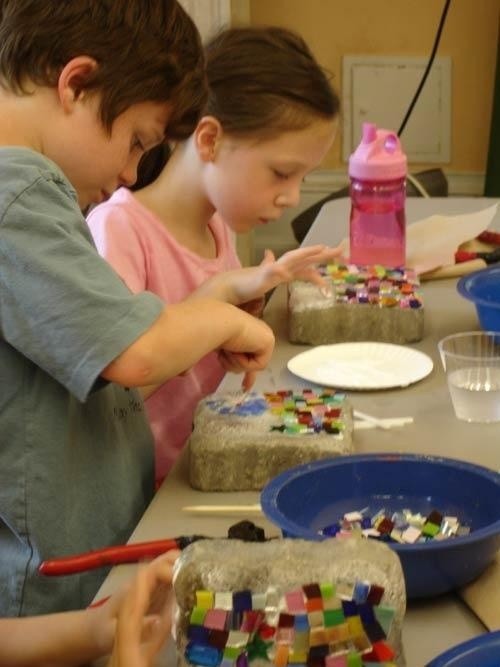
[259,449,500,608]
[456,266,499,343]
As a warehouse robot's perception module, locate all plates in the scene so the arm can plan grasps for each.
[285,339,435,391]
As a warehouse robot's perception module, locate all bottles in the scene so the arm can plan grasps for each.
[346,121,409,270]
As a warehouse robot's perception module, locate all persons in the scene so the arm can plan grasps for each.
[2,544,206,665]
[69,23,352,490]
[2,1,279,625]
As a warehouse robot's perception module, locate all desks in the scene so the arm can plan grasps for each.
[82,193,498,665]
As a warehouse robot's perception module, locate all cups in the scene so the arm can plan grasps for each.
[437,330,500,425]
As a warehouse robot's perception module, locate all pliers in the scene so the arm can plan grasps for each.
[38,534,212,611]
[455,231,500,265]
